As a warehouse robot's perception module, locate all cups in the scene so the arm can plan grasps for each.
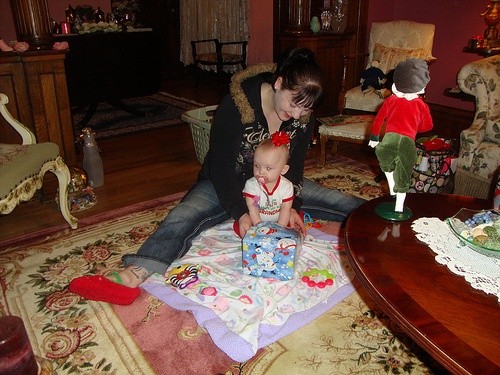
[60,21,71,34]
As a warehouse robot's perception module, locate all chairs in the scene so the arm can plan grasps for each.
[331,19,437,114]
[0,93,78,230]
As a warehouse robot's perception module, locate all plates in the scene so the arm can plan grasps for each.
[447,207,500,256]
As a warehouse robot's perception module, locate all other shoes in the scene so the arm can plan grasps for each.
[68,272,142,305]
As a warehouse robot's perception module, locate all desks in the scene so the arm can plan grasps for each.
[338,192,500,375]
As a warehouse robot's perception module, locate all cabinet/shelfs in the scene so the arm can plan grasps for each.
[0,47,76,165]
[280,33,356,112]
[53,28,157,99]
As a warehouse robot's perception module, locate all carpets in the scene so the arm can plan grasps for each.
[71,92,206,144]
[1,156,431,375]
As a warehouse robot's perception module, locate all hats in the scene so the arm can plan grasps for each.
[57,166,98,213]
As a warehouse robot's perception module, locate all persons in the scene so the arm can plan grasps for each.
[69,49,368,305]
[239,131,294,228]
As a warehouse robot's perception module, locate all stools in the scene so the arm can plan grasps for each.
[0,316,41,375]
[318,114,376,170]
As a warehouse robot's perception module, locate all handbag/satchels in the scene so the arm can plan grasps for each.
[407,133,462,194]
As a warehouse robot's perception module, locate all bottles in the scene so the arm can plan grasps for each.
[80,127,104,187]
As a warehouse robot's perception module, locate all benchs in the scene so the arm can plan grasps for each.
[190,38,247,95]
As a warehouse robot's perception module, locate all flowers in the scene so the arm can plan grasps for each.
[271,129,292,146]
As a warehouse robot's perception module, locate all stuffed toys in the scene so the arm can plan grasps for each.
[360,57,433,212]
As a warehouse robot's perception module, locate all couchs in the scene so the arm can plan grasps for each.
[456,54,500,196]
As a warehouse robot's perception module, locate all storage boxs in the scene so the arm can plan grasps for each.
[181,104,218,165]
